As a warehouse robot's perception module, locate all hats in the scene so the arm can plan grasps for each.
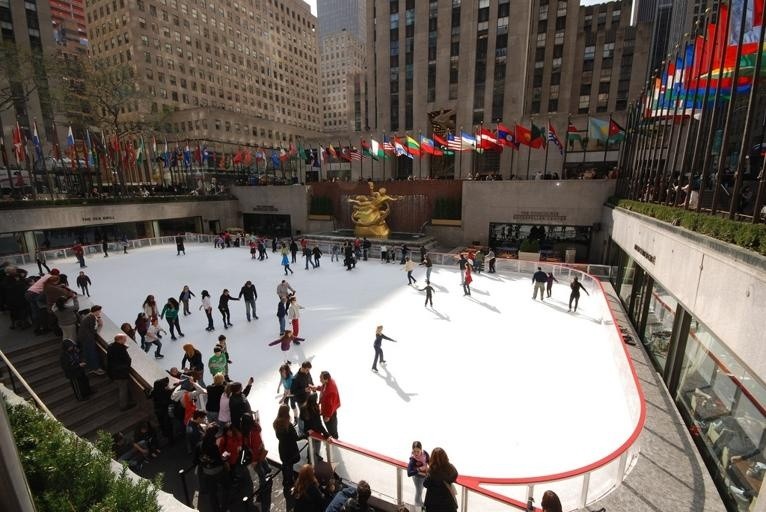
[90,304,102,313]
[154,376,169,392]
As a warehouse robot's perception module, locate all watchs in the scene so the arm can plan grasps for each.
[372,326,397,373]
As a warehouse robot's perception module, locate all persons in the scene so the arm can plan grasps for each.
[175,232,187,255]
[423,447,459,511]
[546,272,560,297]
[117,280,258,356]
[33,248,50,274]
[181,173,226,197]
[120,231,129,254]
[416,281,436,307]
[150,335,272,503]
[71,239,88,268]
[455,246,496,296]
[236,165,619,186]
[400,256,417,285]
[526,490,564,511]
[213,231,371,277]
[103,241,109,257]
[399,242,410,265]
[491,224,567,240]
[345,182,405,215]
[407,441,430,504]
[272,360,374,511]
[643,165,766,223]
[531,267,547,299]
[419,253,433,283]
[381,242,387,264]
[0,261,138,411]
[419,245,425,266]
[276,280,304,337]
[389,243,396,263]
[567,276,589,312]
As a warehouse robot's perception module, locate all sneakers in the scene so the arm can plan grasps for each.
[155,353,164,358]
[380,360,386,363]
[181,310,259,330]
[372,365,379,372]
[171,333,184,340]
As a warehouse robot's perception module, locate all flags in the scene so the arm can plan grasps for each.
[0,118,631,167]
[627,2,765,139]
